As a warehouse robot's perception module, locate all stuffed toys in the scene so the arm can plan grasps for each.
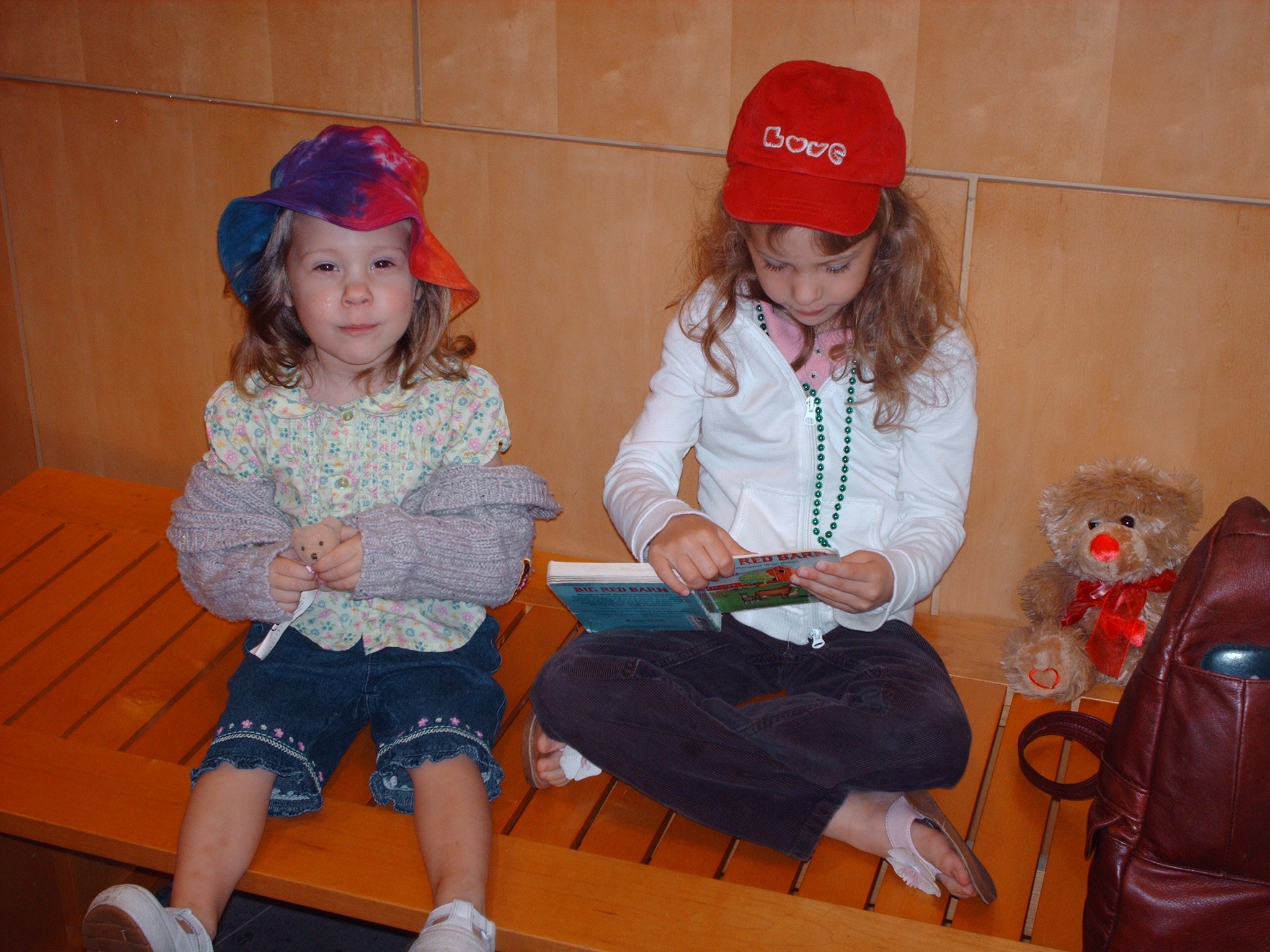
[291,516,343,592]
[999,456,1206,704]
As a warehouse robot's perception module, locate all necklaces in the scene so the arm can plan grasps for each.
[755,298,858,548]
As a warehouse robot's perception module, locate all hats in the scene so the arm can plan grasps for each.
[722,61,908,235]
[216,123,481,326]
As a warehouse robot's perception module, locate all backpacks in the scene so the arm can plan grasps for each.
[1016,498,1270,952]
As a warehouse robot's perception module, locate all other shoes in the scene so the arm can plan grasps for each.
[408,899,497,952]
[82,884,214,952]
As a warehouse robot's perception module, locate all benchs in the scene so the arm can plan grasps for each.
[0,465,1134,952]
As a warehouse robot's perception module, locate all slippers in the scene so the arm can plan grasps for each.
[521,711,603,790]
[886,789,998,904]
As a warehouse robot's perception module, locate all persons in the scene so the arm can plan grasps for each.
[81,126,562,952]
[521,59,998,906]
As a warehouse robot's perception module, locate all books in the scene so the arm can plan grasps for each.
[546,547,842,634]
